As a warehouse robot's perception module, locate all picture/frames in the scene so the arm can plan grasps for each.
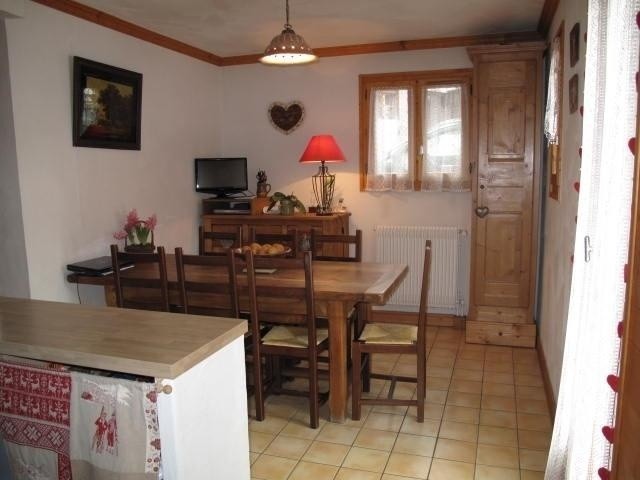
[71,55,143,151]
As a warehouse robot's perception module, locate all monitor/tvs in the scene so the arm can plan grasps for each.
[195,157,248,199]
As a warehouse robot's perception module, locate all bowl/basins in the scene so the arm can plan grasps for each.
[230,248,293,268]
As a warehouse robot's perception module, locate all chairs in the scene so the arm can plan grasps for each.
[352,239,433,422]
[245,249,330,429]
[174,247,271,416]
[110,244,170,312]
[199,224,364,367]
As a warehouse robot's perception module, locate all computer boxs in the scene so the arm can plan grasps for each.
[202,198,248,215]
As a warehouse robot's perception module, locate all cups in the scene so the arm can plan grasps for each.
[257,182,271,198]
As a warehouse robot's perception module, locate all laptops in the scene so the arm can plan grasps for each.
[67,255,133,274]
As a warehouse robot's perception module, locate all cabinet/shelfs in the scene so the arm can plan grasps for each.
[465,41,547,349]
[201,210,352,258]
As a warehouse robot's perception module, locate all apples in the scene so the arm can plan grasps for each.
[242,241,284,255]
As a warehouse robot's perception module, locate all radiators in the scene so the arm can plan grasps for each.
[373,224,468,318]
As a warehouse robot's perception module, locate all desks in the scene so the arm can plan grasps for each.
[66,249,409,424]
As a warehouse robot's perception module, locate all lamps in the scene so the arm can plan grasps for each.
[298,135,347,216]
[256,1,320,66]
